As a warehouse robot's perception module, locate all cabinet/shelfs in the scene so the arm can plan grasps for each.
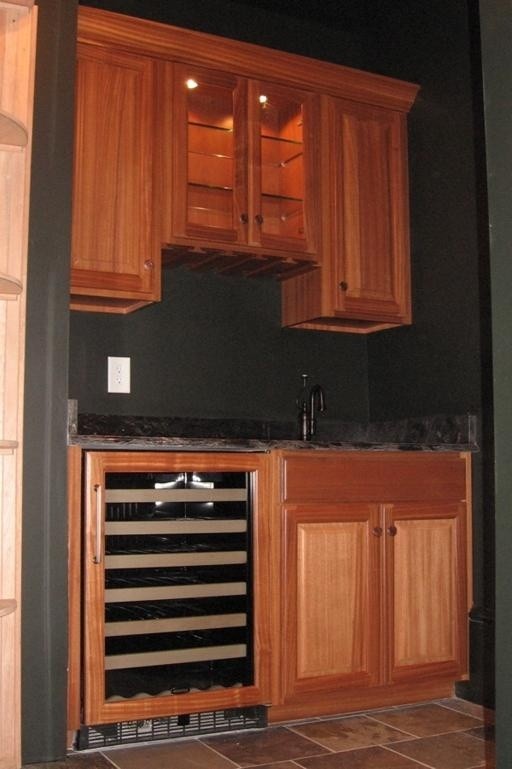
[70,41,167,315]
[1,1,43,767]
[280,97,413,337]
[160,63,324,282]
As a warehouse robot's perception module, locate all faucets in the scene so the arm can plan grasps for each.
[308,383,327,436]
